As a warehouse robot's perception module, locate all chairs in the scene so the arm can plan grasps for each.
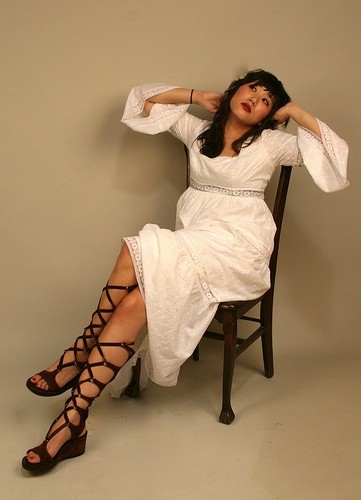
[124,144,292,425]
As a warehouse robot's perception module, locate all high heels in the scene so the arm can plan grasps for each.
[21,341,136,472]
[26,280,140,397]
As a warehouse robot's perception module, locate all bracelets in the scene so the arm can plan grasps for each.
[190,89,195,104]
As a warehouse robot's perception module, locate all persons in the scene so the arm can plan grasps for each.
[21,68,349,472]
